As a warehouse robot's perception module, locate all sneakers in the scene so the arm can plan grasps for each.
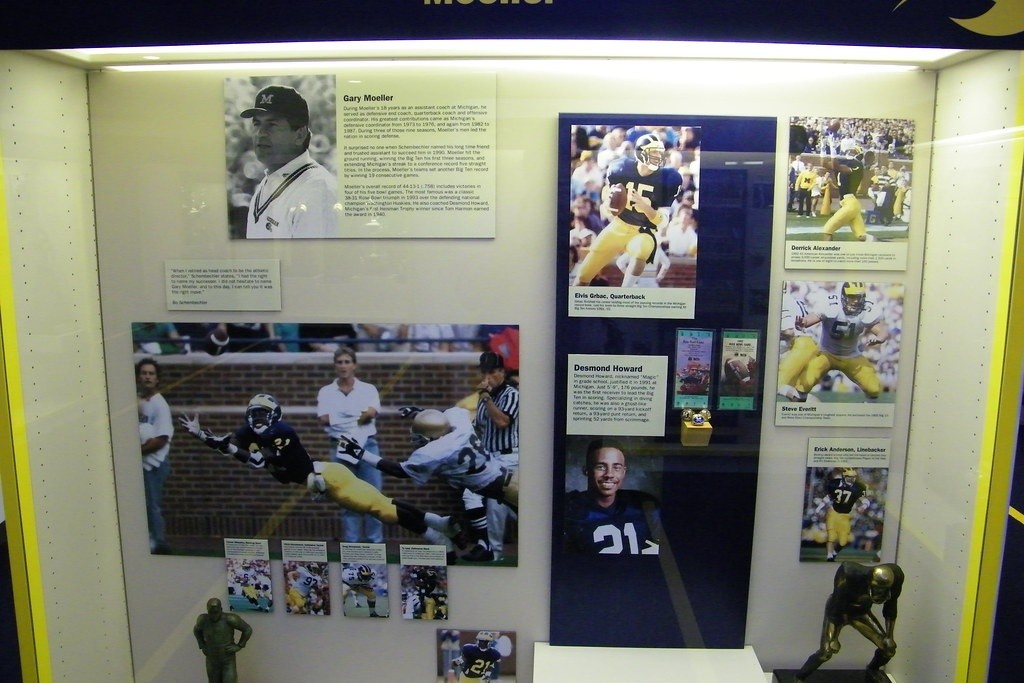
[432,515,494,565]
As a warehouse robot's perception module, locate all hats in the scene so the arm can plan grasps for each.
[240,86,310,122]
[476,351,503,369]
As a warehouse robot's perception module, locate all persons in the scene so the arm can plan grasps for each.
[401,565,448,619]
[283,561,330,614]
[227,558,273,613]
[437,630,502,683]
[789,116,913,242]
[131,322,182,353]
[793,562,904,683]
[179,324,520,565]
[570,126,700,288]
[804,466,887,561]
[565,438,649,576]
[194,598,252,683]
[341,564,379,616]
[135,358,174,555]
[241,85,337,238]
[777,281,904,402]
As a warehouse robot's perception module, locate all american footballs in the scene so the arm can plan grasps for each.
[204,327,231,357]
[724,357,752,386]
[610,182,628,216]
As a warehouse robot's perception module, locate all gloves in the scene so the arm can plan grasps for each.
[398,406,424,419]
[204,428,233,450]
[178,412,203,438]
[335,435,366,461]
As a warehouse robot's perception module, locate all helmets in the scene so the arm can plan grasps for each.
[412,409,450,440]
[843,467,858,488]
[841,281,866,316]
[476,630,493,641]
[304,562,319,575]
[844,143,864,161]
[245,393,284,435]
[634,134,667,171]
[357,564,375,584]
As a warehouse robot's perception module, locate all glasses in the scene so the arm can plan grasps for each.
[691,369,697,372]
[335,359,355,364]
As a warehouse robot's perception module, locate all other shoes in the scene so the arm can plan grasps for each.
[827,553,833,561]
[796,211,817,217]
[370,611,379,617]
[833,550,838,557]
